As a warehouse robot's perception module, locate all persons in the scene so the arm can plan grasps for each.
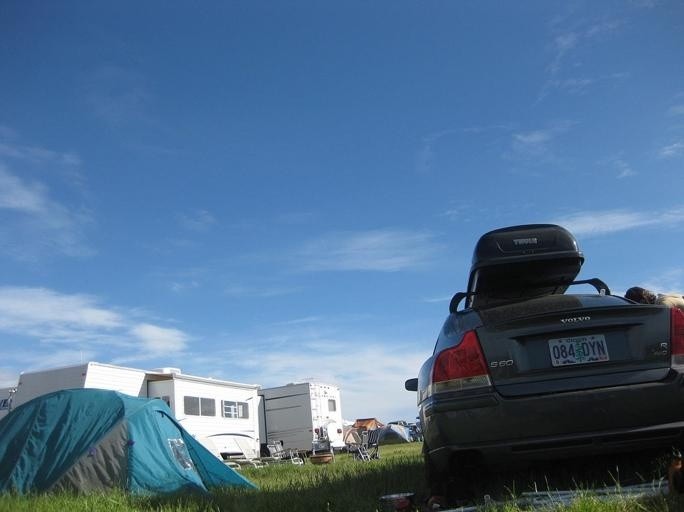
[412,425,423,442]
[624,286,684,313]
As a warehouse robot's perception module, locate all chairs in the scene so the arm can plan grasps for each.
[215,451,263,471]
[267,440,308,466]
[345,428,382,462]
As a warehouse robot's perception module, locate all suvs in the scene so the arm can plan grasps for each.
[404,279,683,507]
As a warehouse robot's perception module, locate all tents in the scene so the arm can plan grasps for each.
[0,387,259,499]
